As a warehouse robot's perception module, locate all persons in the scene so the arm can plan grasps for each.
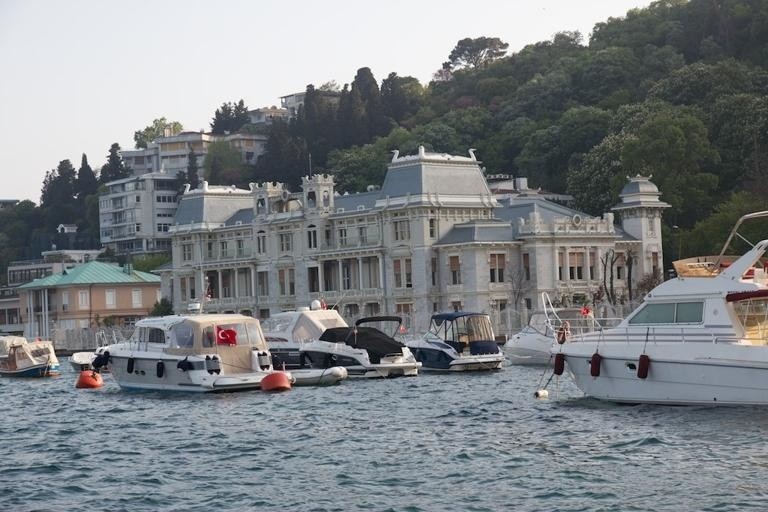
[92,352,112,374]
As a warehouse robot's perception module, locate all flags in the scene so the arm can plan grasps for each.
[217,326,237,345]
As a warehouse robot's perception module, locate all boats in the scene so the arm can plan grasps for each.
[0,336,62,379]
[402,312,505,373]
[541,210,768,412]
[263,295,425,380]
[68,331,110,375]
[502,306,594,366]
[92,310,295,395]
